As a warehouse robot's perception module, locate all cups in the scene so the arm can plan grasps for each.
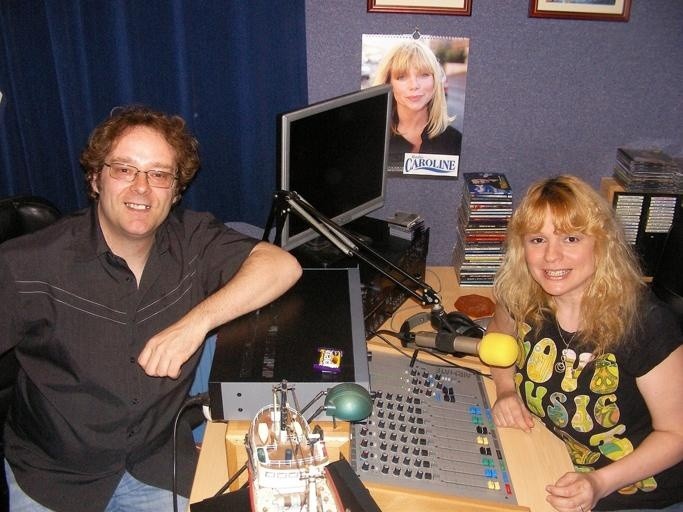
[103,162,178,189]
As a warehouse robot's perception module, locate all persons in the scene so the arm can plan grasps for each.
[1,108,305,512]
[375,39,461,168]
[483,173,682,512]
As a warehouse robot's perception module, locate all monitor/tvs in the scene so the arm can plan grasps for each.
[390,211,421,228]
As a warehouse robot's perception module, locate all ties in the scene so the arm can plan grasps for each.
[275,83,393,268]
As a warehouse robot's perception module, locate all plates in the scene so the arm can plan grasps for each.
[599,176,683,283]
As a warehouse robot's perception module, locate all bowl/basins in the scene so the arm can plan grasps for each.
[552,312,585,374]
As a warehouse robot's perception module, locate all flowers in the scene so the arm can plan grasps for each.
[413,330,519,368]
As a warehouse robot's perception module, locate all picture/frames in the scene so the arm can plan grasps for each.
[186,266,588,512]
